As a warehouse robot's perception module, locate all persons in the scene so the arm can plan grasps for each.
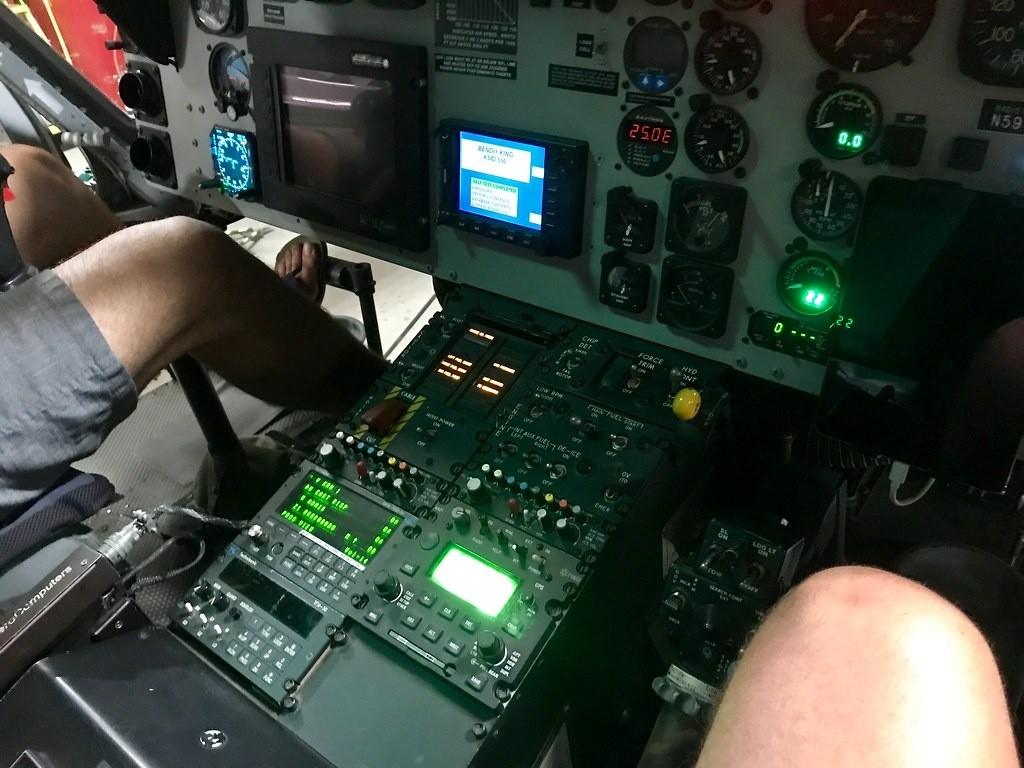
[694,564,1024,767]
[1,144,393,482]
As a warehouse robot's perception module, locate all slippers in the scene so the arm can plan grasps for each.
[273,236,328,306]
